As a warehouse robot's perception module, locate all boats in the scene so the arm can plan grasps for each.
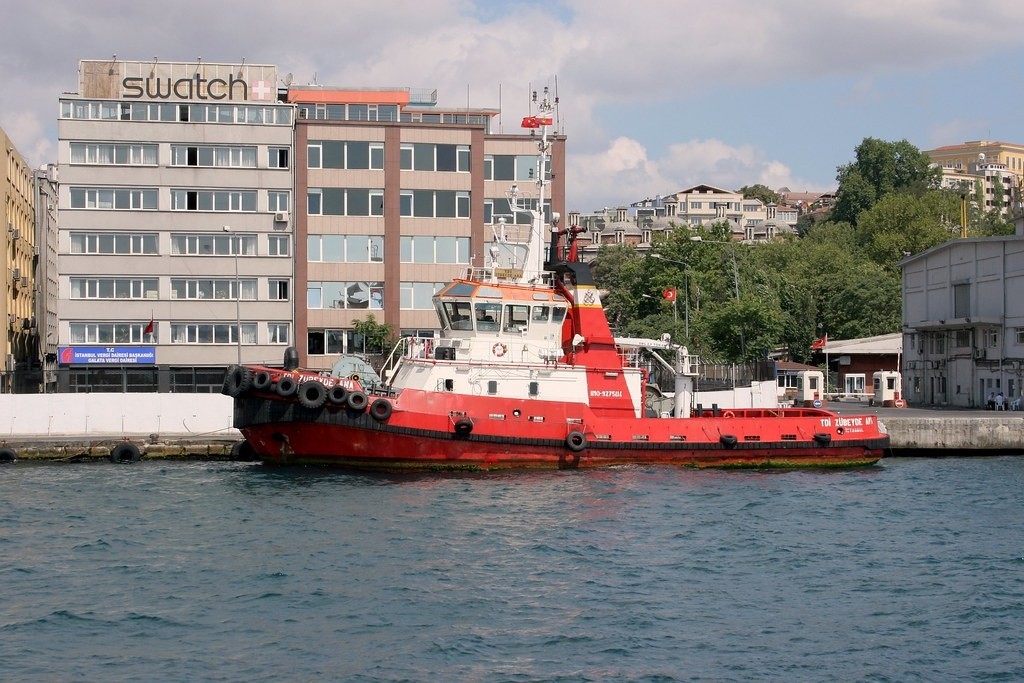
[221,72,891,471]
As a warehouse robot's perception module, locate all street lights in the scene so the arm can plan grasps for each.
[642,286,677,338]
[690,235,748,385]
[223,225,243,365]
[650,253,689,340]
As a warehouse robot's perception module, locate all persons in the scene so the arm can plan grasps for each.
[987,391,1007,410]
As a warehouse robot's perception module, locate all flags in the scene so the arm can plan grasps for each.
[521,116,553,128]
[812,336,825,349]
[144,321,154,334]
[664,289,675,300]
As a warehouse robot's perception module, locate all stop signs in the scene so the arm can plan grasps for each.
[895,400,903,408]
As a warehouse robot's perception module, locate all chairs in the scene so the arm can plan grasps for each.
[1009,398,1021,411]
[995,401,1004,411]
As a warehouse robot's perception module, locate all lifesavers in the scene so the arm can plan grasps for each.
[719,434,738,445]
[492,342,508,358]
[220,365,393,421]
[567,431,587,452]
[454,419,473,435]
[814,433,832,443]
[723,410,736,418]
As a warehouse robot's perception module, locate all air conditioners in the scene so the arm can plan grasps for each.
[11,313,17,323]
[33,282,38,292]
[7,354,14,371]
[22,276,28,287]
[909,362,914,369]
[916,349,922,354]
[15,268,21,279]
[13,228,21,239]
[975,349,984,359]
[8,222,13,232]
[275,213,288,223]
[35,246,40,255]
